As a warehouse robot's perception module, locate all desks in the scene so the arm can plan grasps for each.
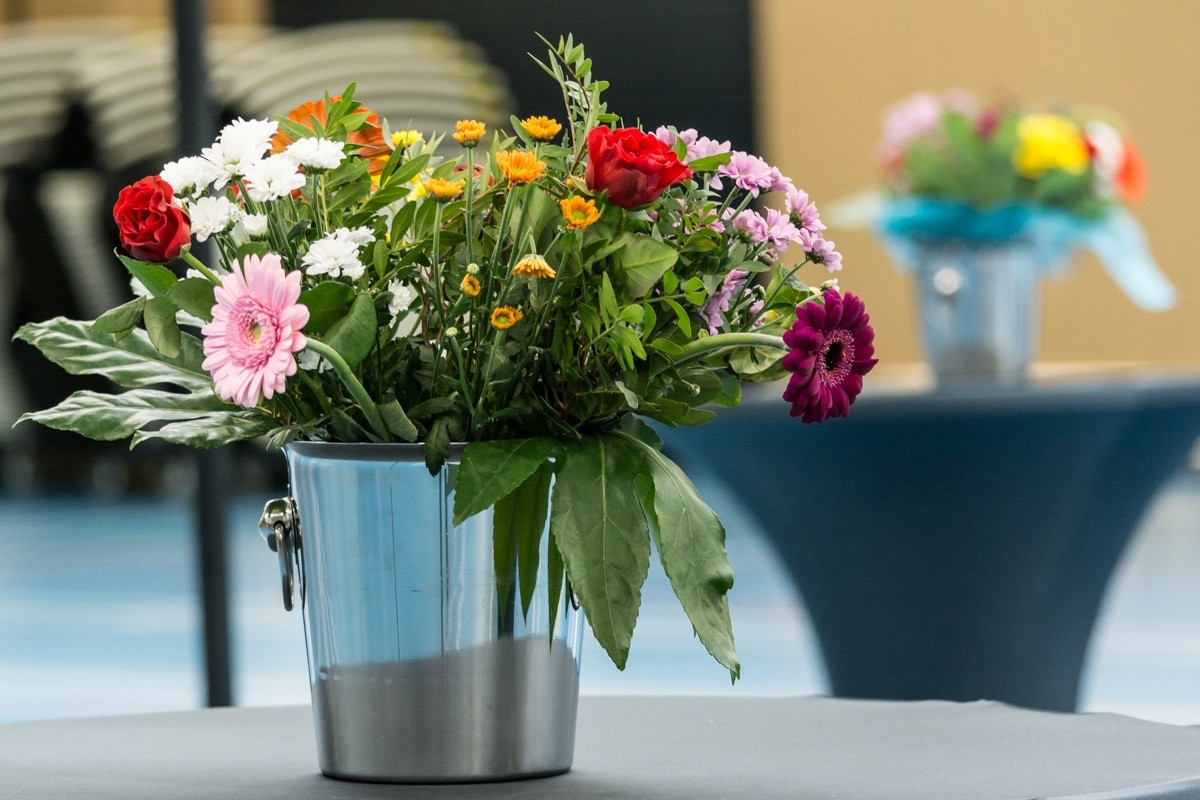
[635,354,1200,711]
[0,690,1200,800]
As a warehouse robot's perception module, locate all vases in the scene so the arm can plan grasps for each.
[913,238,1042,394]
[257,435,586,783]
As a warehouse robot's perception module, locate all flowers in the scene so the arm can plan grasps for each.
[879,83,1154,219]
[11,29,885,684]
